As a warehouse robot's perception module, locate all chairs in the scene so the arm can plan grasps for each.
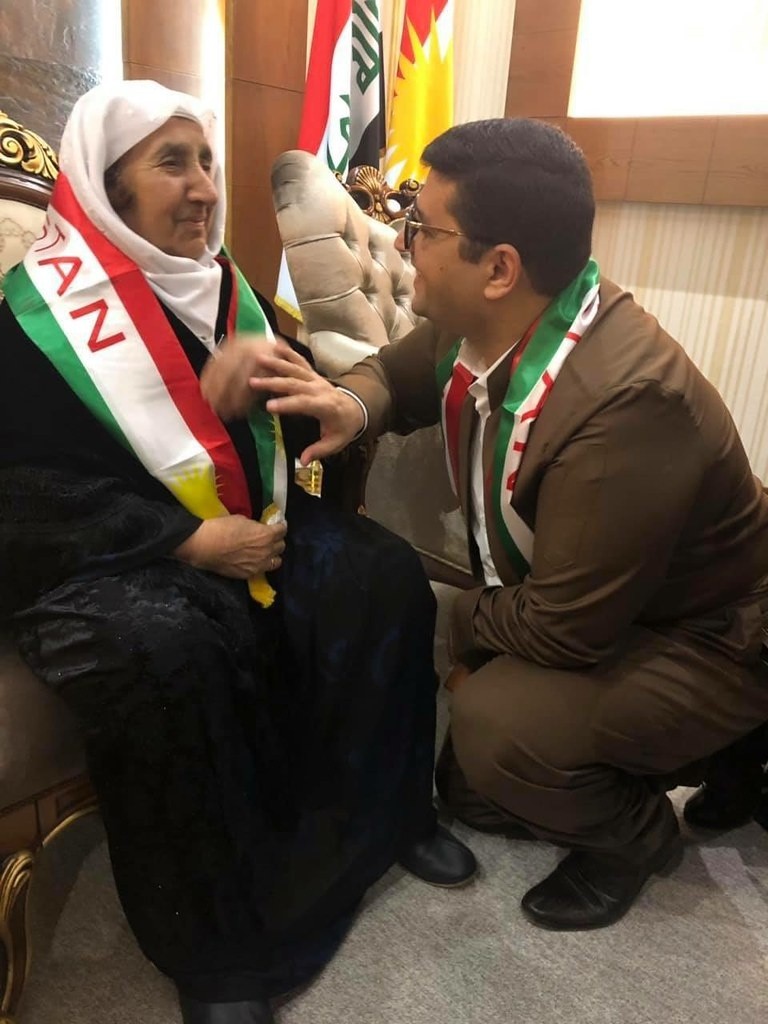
[270,149,472,591]
[0,109,104,1024]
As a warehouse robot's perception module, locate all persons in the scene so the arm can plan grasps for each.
[0,81,479,1023]
[248,113,768,930]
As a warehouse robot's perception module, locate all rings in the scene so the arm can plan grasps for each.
[271,559,274,569]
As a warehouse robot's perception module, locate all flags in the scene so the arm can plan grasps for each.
[274,0,455,323]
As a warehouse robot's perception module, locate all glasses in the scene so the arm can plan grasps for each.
[403,206,527,257]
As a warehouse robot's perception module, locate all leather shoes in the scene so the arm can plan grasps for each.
[519,814,685,932]
[682,768,767,838]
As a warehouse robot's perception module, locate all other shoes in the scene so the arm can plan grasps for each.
[181,995,273,1024]
[399,824,480,888]
